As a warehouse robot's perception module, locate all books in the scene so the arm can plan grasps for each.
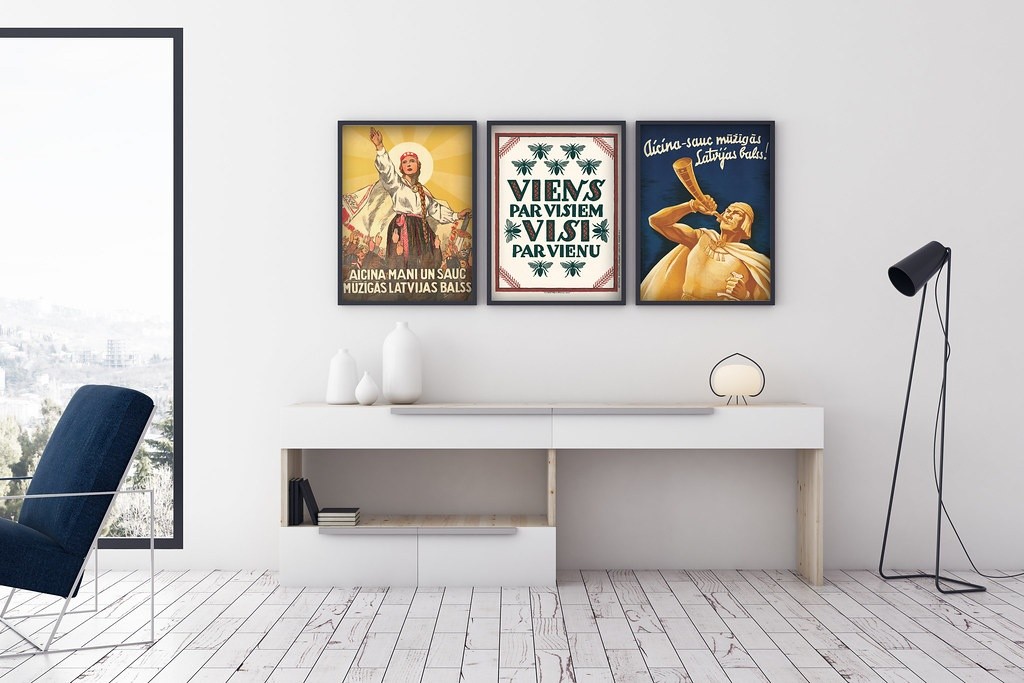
[287,478,359,527]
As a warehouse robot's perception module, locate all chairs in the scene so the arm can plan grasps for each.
[0,383,158,661]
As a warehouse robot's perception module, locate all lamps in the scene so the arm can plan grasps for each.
[884,238,986,596]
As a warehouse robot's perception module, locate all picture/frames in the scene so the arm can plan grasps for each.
[337,119,476,305]
[486,120,627,304]
[3,26,186,553]
[633,120,775,305]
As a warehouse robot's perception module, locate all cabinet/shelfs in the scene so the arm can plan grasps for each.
[273,400,825,588]
